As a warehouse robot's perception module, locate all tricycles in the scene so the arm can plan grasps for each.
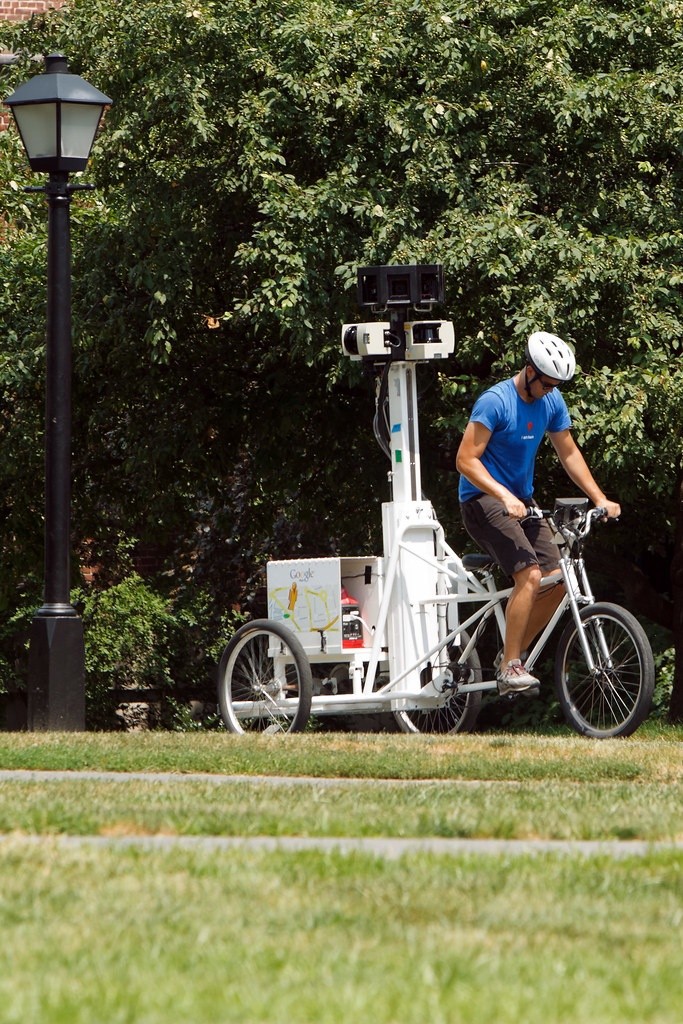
[216,510,659,740]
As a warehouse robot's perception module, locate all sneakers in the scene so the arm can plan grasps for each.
[493,648,540,697]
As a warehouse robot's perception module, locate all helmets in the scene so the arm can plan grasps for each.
[525,331,576,380]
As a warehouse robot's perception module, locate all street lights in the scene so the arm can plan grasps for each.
[0,55,116,734]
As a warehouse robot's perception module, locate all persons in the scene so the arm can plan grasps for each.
[456,331,622,698]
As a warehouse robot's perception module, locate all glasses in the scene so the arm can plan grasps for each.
[538,378,561,391]
